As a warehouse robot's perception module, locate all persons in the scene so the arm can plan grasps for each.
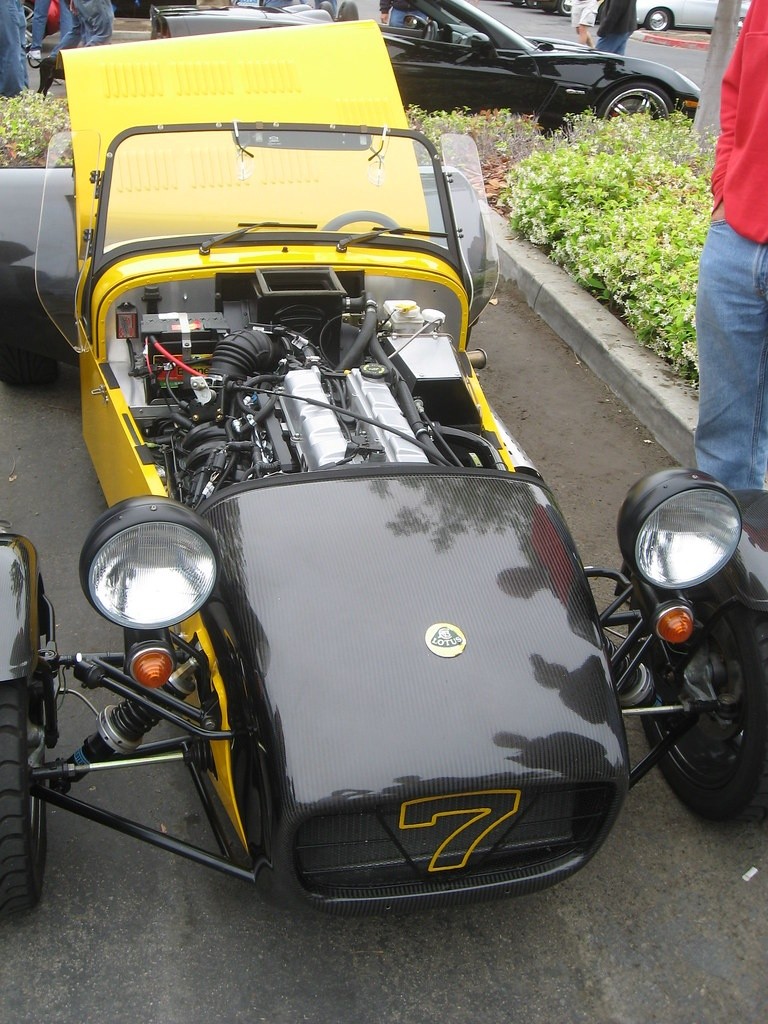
[0,0,339,99]
[571,0,599,48]
[695,0,768,493]
[595,0,637,56]
[379,0,442,30]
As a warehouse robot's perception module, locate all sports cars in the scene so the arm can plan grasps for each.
[147,0,701,141]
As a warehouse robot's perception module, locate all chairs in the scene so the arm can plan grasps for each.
[336,1,358,21]
[321,1,336,20]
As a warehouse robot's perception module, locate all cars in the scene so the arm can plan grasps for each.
[506,0,752,38]
[2,21,768,924]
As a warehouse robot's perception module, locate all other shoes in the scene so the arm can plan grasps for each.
[25,50,41,60]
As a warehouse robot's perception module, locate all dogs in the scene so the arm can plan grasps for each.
[27,55,64,101]
